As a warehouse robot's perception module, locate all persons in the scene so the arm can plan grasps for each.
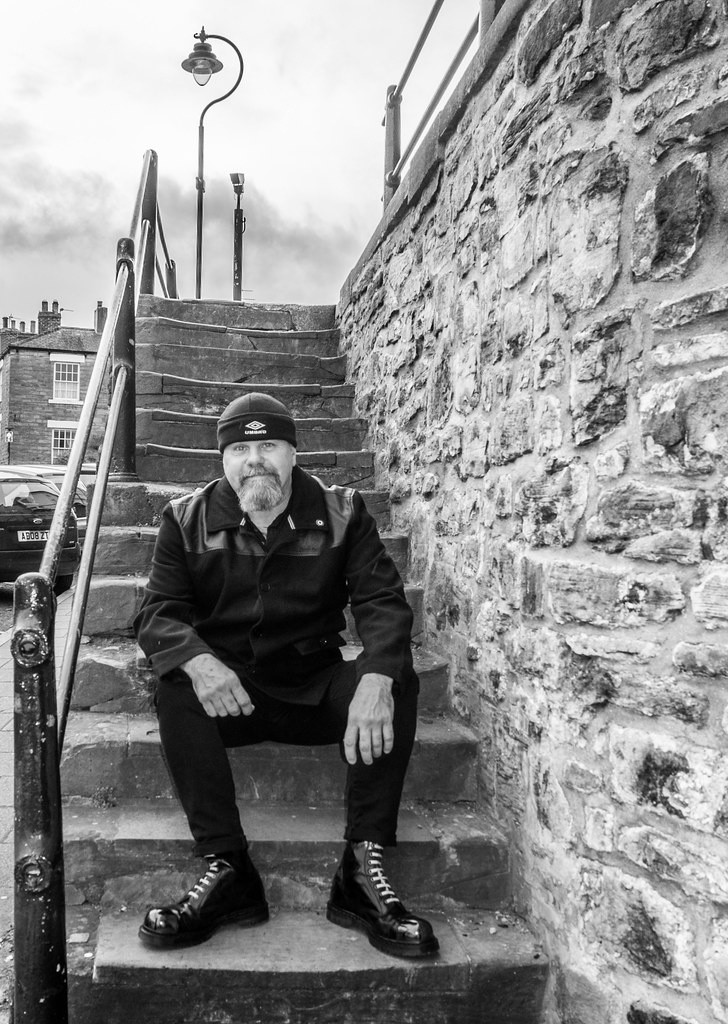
[132,393,440,957]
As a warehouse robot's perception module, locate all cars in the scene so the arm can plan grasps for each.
[0,468,83,597]
[3,460,98,556]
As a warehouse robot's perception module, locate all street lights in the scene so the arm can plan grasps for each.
[229,173,246,301]
[180,23,245,295]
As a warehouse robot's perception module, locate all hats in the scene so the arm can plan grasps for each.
[216,392,297,449]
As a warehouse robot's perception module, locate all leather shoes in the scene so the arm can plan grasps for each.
[326,840,440,957]
[136,851,269,950]
